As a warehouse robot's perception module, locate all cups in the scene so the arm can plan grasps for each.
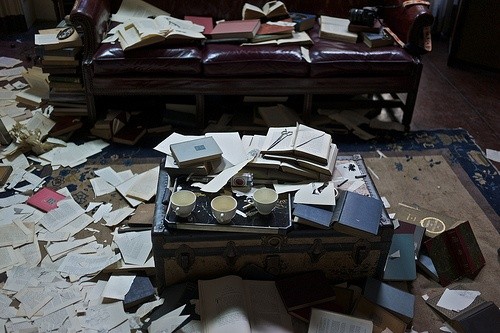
[169,190,197,218]
[253,187,279,214]
[211,196,237,224]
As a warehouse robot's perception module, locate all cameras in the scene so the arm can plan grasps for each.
[348,6,379,29]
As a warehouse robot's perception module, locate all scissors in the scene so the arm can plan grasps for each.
[267,128,292,150]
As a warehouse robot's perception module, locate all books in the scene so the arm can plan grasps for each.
[0,0,500,333]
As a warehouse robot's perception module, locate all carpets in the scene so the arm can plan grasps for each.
[1,128,500,332]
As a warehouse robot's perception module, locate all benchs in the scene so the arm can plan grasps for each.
[70,0,435,131]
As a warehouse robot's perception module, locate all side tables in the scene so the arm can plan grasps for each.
[151,153,393,291]
